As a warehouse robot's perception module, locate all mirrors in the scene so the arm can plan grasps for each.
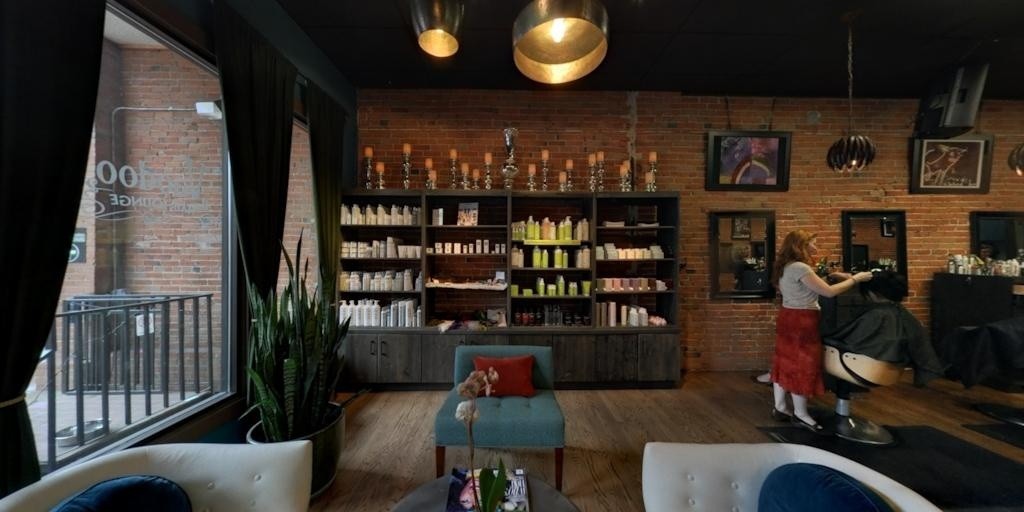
[969,210,1024,262]
[708,209,777,301]
[841,208,909,297]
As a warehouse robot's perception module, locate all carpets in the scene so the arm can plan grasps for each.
[962,422,1024,455]
[754,424,1024,512]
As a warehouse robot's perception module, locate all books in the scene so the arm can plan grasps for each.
[446,467,530,512]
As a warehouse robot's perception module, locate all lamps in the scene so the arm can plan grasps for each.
[827,14,877,173]
[513,0,609,85]
[1008,142,1024,177]
[408,0,468,58]
[110,99,223,297]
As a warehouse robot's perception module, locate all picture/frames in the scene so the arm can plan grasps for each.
[731,216,752,241]
[909,134,995,194]
[705,128,793,192]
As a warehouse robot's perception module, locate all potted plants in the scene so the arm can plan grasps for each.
[238,226,354,498]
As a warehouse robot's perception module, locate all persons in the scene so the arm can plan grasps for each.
[771,229,873,434]
[751,270,925,386]
[449,469,482,511]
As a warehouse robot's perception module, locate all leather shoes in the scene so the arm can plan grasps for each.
[751,375,774,388]
[791,414,828,436]
[772,409,793,421]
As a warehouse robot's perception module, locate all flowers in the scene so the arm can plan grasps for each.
[455,367,500,512]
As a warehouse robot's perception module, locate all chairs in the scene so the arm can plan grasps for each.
[818,344,905,445]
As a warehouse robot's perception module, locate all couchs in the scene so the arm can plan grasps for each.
[0,438,312,512]
[434,345,565,492]
[642,441,945,512]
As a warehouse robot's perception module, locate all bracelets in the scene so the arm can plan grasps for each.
[850,276,858,285]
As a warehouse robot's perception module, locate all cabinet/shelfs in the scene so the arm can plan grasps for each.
[336,329,422,391]
[336,190,680,329]
[509,329,595,391]
[423,329,509,391]
[595,328,682,390]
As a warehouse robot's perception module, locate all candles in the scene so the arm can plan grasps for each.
[559,171,567,184]
[376,161,385,173]
[403,142,411,154]
[425,158,432,169]
[485,152,492,161]
[528,163,536,175]
[566,159,573,169]
[648,151,656,160]
[541,149,549,159]
[449,149,457,159]
[429,170,437,181]
[365,147,372,158]
[623,160,630,171]
[597,151,605,162]
[620,164,628,178]
[461,163,468,172]
[646,172,655,183]
[473,169,479,178]
[589,153,595,167]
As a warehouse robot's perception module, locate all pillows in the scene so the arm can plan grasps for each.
[48,474,193,511]
[472,353,536,399]
[757,463,895,512]
